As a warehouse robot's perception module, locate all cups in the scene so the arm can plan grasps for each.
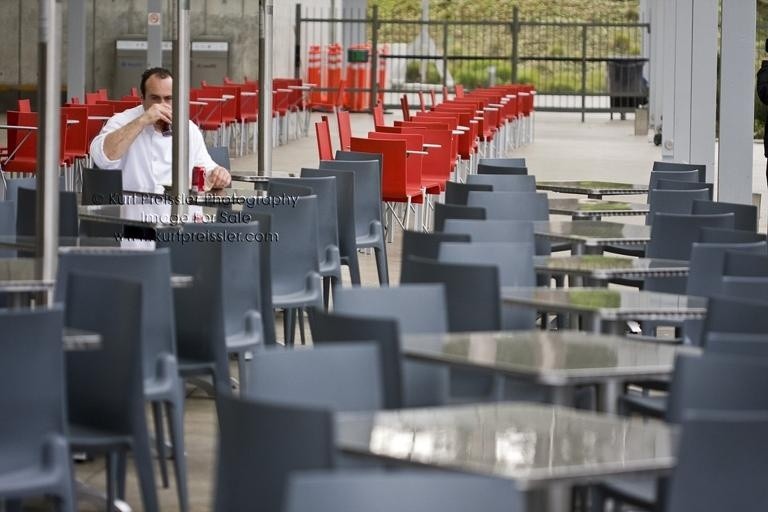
[158,120,171,137]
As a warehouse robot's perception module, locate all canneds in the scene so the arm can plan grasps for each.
[191,166,206,195]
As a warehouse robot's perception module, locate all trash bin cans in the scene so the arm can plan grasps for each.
[608,58,650,109]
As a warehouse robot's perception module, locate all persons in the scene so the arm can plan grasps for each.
[757,38,768,188]
[90,67,232,250]
[605,39,645,90]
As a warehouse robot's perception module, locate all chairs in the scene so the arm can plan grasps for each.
[606,62,648,121]
